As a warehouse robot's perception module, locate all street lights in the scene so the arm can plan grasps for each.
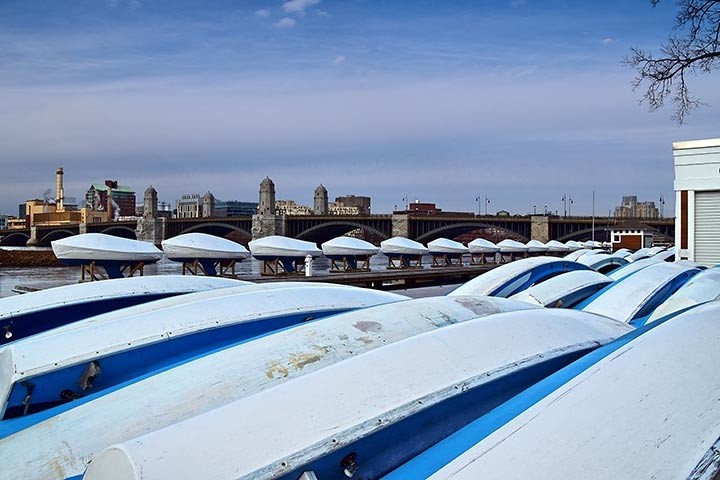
[569,197,573,217]
[476,196,480,215]
[485,198,490,215]
[562,193,567,218]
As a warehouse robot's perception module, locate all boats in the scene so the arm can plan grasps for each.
[427,237,469,264]
[320,236,381,269]
[381,236,429,268]
[0,239,720,480]
[525,239,549,256]
[247,235,323,273]
[51,232,163,278]
[545,240,570,257]
[467,238,501,264]
[496,238,529,261]
[160,232,251,276]
